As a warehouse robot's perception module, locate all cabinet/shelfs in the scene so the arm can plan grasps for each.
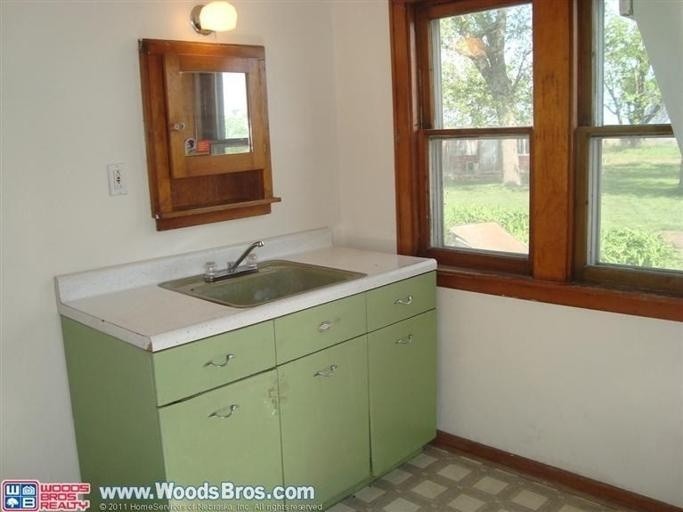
[53,227,438,510]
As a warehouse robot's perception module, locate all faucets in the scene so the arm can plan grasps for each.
[203,239,265,282]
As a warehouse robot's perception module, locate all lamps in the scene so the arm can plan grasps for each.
[189,0,238,37]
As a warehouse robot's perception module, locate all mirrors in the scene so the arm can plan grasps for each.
[162,52,267,180]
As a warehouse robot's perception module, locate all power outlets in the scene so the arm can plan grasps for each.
[106,162,130,196]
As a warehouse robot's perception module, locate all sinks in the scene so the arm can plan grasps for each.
[158,260,368,309]
